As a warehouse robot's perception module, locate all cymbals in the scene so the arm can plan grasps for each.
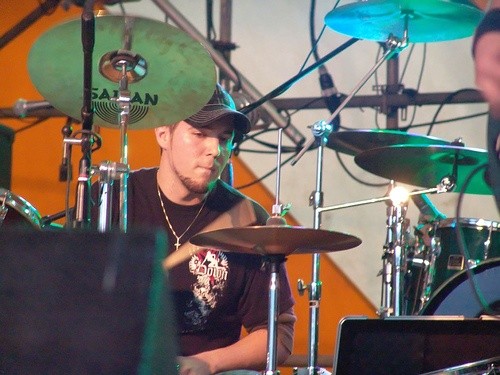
[323,0,486,43]
[354,145,496,196]
[327,128,465,157]
[26,15,217,131]
[189,224,362,255]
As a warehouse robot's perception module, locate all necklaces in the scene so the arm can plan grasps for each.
[157,184,208,251]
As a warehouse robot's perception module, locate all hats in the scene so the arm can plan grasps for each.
[184,84,250,133]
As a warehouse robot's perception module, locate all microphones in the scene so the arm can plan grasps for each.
[318,62,339,114]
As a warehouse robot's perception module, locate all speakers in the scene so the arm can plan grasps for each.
[0,226,182,375]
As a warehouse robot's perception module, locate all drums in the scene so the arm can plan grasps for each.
[0,188,42,230]
[417,258,500,316]
[400,216,500,317]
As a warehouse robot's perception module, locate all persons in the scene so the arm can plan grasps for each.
[70,84,296,375]
[472,7,500,212]
